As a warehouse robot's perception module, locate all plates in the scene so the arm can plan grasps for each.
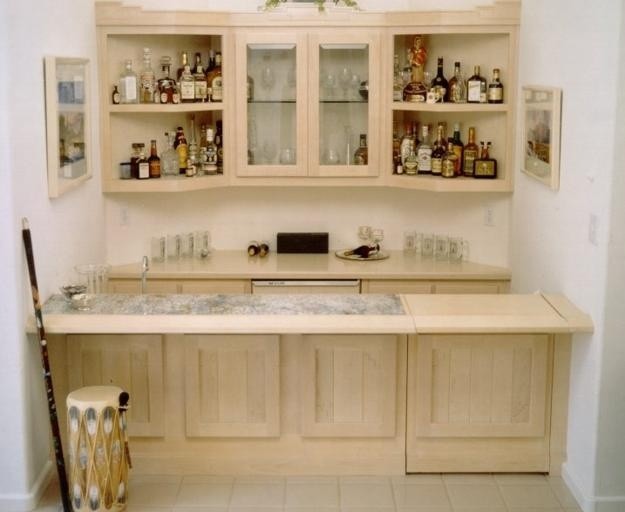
[336,248,390,259]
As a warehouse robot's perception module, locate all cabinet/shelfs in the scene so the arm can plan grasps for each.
[238,27,381,178]
[100,29,234,192]
[256,281,360,295]
[410,338,551,476]
[364,279,509,294]
[385,27,515,192]
[100,277,251,294]
[61,335,404,477]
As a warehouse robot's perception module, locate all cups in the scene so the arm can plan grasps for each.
[428,90,439,104]
[435,235,448,260]
[449,238,462,264]
[338,67,353,101]
[263,143,275,162]
[372,231,383,249]
[280,149,295,164]
[349,73,361,102]
[452,68,469,103]
[181,234,192,257]
[324,149,340,165]
[402,232,417,256]
[193,233,209,258]
[149,237,165,264]
[358,226,370,245]
[166,235,180,260]
[260,67,273,98]
[208,87,213,103]
[422,234,433,258]
[322,71,337,98]
[199,87,206,103]
[420,71,433,101]
[396,71,411,101]
[160,130,180,178]
[439,87,446,104]
[76,264,110,302]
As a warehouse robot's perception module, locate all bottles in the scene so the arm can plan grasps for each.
[431,57,449,102]
[399,121,416,164]
[247,241,259,256]
[453,123,464,175]
[139,49,156,103]
[395,155,404,175]
[112,85,120,104]
[118,160,131,179]
[442,138,459,178]
[185,158,194,178]
[473,141,497,179]
[448,62,467,103]
[393,54,404,101]
[467,66,487,102]
[431,130,445,176]
[214,120,224,175]
[176,52,192,80]
[148,140,161,179]
[207,52,223,102]
[119,58,140,104]
[416,125,433,174]
[172,127,189,175]
[403,140,419,175]
[204,49,216,75]
[178,64,195,103]
[354,134,369,165]
[156,56,178,105]
[130,142,147,179]
[161,132,180,177]
[188,114,201,169]
[136,152,150,180]
[191,53,206,75]
[463,128,480,177]
[434,123,448,152]
[259,241,270,256]
[488,68,504,104]
[344,242,380,257]
[202,125,218,176]
[193,64,207,102]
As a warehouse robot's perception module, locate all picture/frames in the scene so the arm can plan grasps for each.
[44,56,94,199]
[521,84,562,190]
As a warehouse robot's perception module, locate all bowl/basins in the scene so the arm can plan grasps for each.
[59,284,85,302]
[73,295,93,310]
